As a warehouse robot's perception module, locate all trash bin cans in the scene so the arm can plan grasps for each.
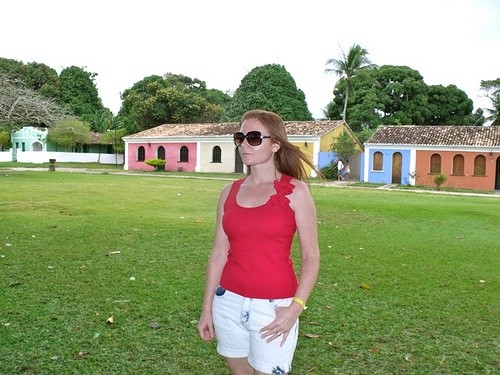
[50,159,56,163]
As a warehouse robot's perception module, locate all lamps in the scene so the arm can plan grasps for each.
[304,141,308,147]
[489,150,494,159]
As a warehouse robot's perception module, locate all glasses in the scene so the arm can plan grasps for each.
[233,131,271,147]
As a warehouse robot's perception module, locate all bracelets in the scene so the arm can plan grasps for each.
[293,297,307,310]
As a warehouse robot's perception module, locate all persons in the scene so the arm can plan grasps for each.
[336,158,352,182]
[196,110,320,375]
